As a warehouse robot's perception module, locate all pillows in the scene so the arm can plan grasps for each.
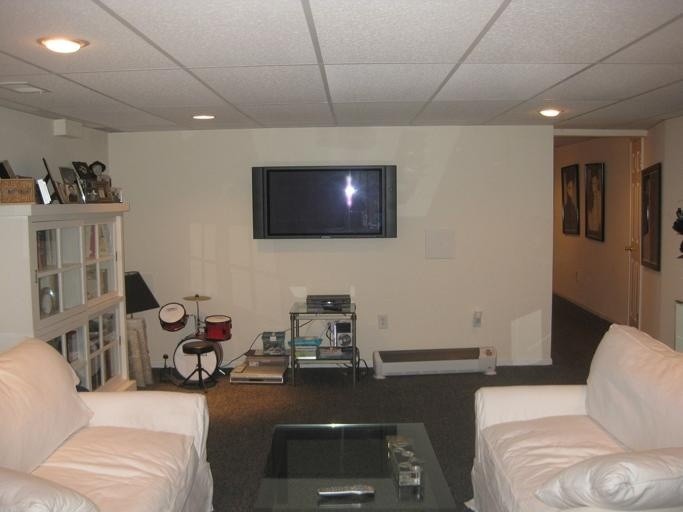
[532,444,683,511]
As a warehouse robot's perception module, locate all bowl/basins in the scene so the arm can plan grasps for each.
[320,299,334,309]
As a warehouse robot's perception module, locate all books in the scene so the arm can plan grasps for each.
[36,224,116,392]
[290,335,342,361]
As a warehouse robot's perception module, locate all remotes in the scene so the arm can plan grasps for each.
[317,484,375,496]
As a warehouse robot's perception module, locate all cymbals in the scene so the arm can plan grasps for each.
[183,295,210,301]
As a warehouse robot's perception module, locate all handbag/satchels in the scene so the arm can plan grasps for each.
[262,332,284,350]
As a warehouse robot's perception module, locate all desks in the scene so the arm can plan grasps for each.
[253,422,455,512]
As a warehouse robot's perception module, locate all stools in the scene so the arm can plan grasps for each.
[176,342,218,394]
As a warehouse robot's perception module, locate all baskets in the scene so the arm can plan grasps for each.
[0,178,40,204]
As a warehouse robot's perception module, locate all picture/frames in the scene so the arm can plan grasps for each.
[584,161,604,242]
[561,164,580,235]
[640,162,661,272]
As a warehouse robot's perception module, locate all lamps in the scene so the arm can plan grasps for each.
[124,270,160,318]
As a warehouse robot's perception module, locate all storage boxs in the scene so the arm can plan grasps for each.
[0,176,35,202]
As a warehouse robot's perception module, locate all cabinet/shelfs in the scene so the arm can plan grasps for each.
[0,296,128,393]
[287,302,356,388]
[0,202,136,330]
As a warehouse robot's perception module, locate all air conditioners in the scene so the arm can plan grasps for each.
[371,344,498,380]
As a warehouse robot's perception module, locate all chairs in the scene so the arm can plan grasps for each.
[0,337,214,511]
[463,324,682,512]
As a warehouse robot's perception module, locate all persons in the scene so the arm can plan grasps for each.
[66,183,80,203]
[564,170,580,231]
[588,168,603,233]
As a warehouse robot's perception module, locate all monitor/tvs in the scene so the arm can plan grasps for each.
[252,165,397,239]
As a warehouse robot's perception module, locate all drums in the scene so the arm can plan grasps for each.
[172,332,223,384]
[159,302,187,332]
[205,315,233,341]
